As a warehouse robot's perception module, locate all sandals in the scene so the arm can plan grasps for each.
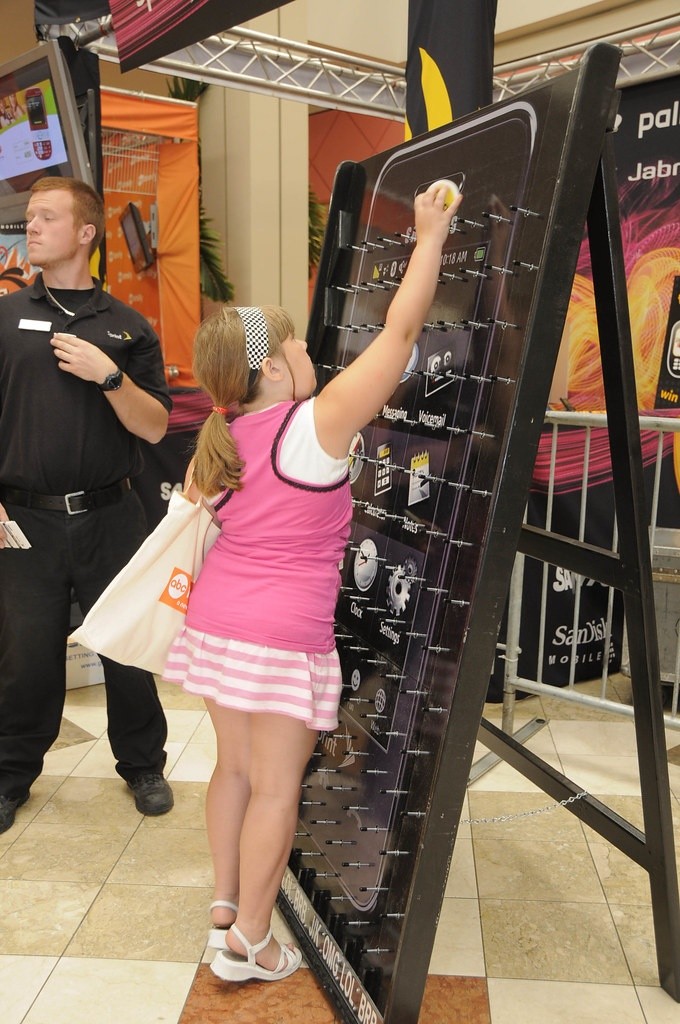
[208,923,302,982]
[208,900,238,952]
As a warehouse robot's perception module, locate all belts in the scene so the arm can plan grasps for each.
[0,477,140,513]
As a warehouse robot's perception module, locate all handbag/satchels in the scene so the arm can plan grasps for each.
[68,489,222,676]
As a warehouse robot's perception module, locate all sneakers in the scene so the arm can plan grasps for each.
[127,771,174,816]
[0,792,30,833]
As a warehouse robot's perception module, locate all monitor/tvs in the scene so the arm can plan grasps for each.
[119,202,156,273]
[0,39,98,236]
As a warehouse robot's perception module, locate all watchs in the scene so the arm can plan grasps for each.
[100,367,123,392]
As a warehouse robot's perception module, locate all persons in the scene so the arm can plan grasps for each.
[162,176,463,980]
[0,177,175,831]
